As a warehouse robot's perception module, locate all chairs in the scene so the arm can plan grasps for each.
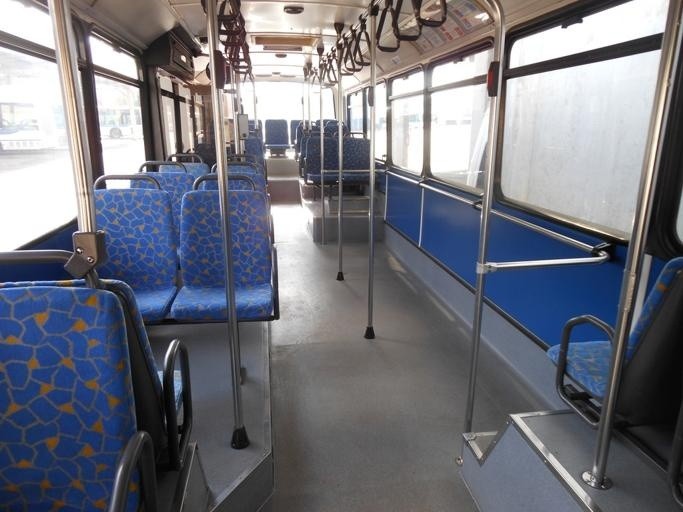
[86,120,281,325]
[0,280,195,512]
[546,256,681,429]
[264,117,381,199]
